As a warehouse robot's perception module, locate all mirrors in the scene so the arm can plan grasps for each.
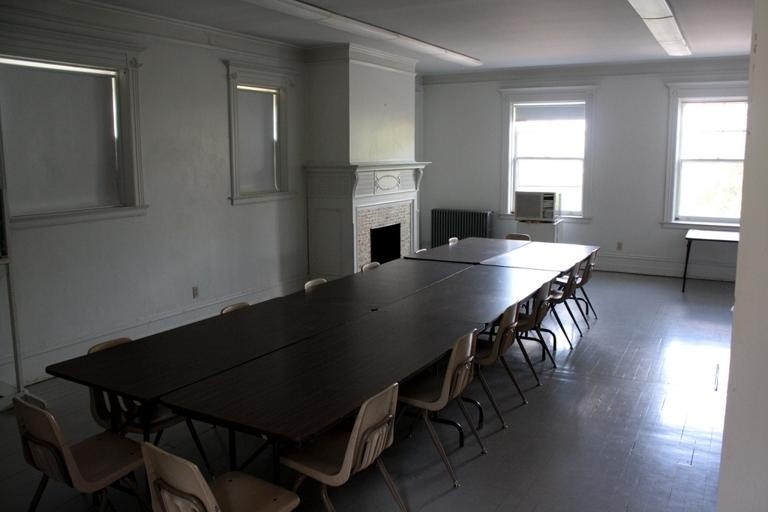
[225,58,295,206]
[0,54,150,228]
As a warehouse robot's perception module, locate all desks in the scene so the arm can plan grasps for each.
[374,264,562,324]
[682,229,740,293]
[404,237,537,263]
[480,241,600,273]
[46,235,602,425]
[279,258,475,311]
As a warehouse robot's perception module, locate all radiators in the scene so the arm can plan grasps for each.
[431,208,492,247]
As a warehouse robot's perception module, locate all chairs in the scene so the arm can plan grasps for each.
[524,263,583,350]
[13,396,143,512]
[398,329,487,487]
[557,249,598,329]
[491,282,558,386]
[362,261,381,272]
[304,278,328,290]
[505,233,531,241]
[89,337,216,480]
[476,304,529,429]
[141,441,301,512]
[281,383,407,512]
[221,302,249,315]
